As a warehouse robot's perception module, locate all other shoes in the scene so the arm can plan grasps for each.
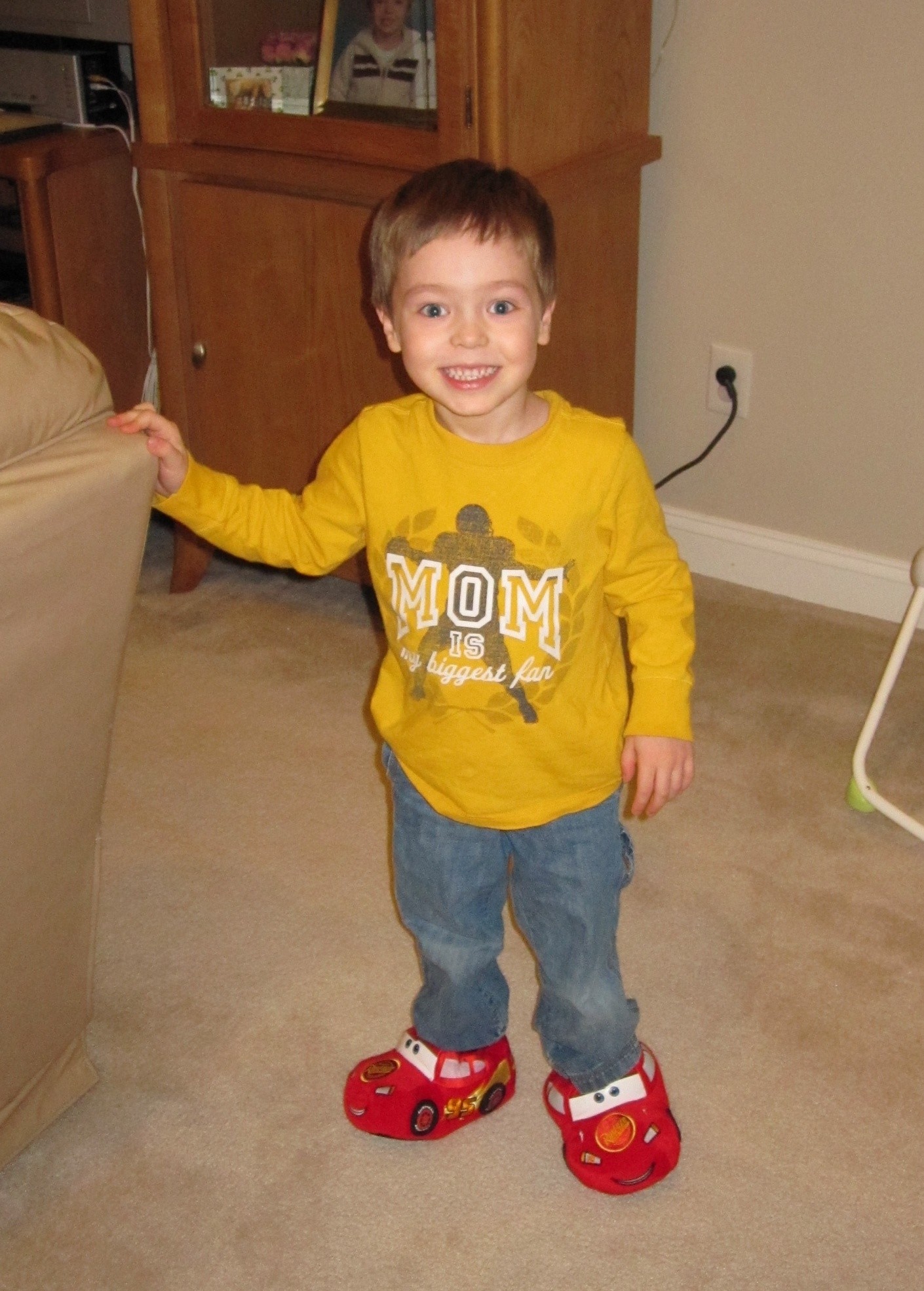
[341,1028,517,1141]
[543,1040,681,1193]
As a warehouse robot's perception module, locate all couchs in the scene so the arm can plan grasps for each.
[0,304,158,1165]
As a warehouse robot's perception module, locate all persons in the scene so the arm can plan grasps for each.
[328,0,437,110]
[106,157,697,1196]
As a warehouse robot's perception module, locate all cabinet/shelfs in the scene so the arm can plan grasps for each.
[130,0,661,596]
[1,130,155,415]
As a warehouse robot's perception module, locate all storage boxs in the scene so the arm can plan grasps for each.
[208,67,315,115]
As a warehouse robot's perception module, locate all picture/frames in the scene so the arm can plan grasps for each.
[313,0,438,117]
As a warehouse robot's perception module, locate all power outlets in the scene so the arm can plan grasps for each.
[707,345,753,419]
